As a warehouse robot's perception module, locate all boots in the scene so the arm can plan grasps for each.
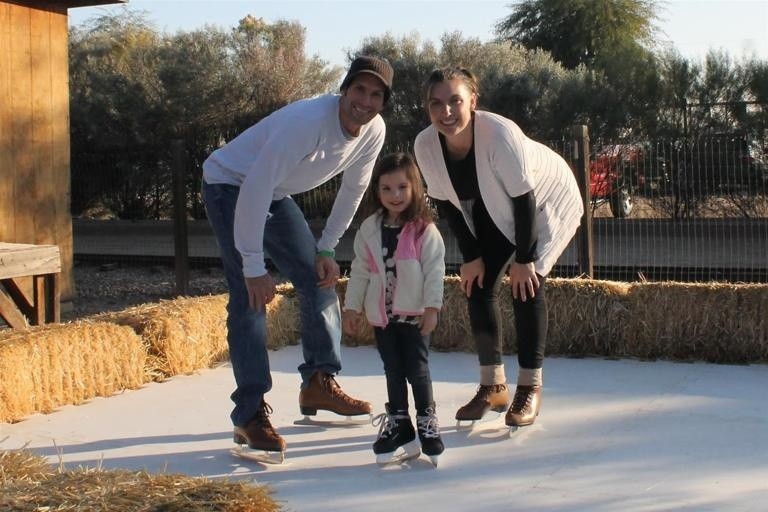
[416,403,444,457]
[373,403,416,454]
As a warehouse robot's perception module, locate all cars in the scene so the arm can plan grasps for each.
[588,131,768,219]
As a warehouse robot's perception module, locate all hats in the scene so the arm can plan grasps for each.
[341,55,394,96]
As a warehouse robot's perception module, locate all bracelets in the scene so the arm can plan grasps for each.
[314,248,336,258]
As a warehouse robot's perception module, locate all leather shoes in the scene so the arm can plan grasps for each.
[234,400,285,452]
[504,385,542,426]
[454,385,510,419]
[299,372,373,417]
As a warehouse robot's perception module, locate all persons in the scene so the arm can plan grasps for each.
[200,54,397,454]
[339,150,449,456]
[411,65,588,429]
